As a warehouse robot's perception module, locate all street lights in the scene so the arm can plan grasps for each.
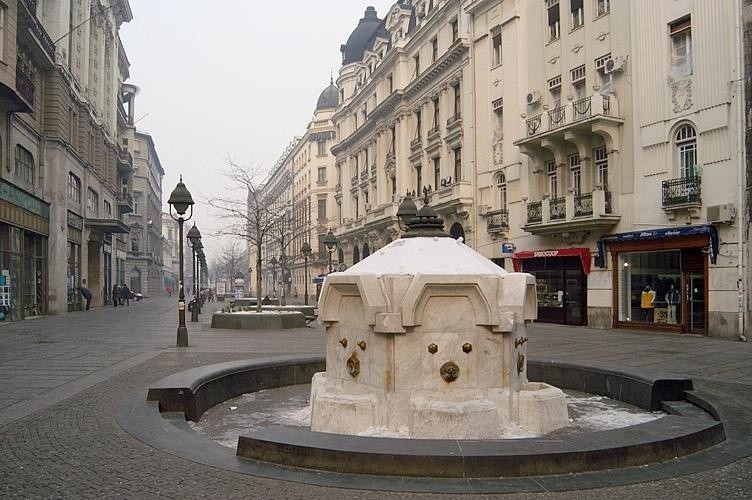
[187,222,209,322]
[323,226,338,273]
[300,241,312,305]
[395,187,419,232]
[248,266,253,297]
[270,254,278,298]
[168,171,194,348]
[279,252,288,306]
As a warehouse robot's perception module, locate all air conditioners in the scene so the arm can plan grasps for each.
[524,89,541,106]
[603,55,623,76]
[706,203,736,225]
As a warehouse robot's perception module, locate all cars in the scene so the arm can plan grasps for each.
[129,290,143,301]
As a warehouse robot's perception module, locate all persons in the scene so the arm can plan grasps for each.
[167,285,172,296]
[294,287,297,297]
[122,284,130,306]
[207,288,212,302]
[117,287,122,305]
[272,289,276,298]
[640,283,657,322]
[665,284,681,324]
[77,287,93,312]
[249,288,252,297]
[112,284,117,307]
